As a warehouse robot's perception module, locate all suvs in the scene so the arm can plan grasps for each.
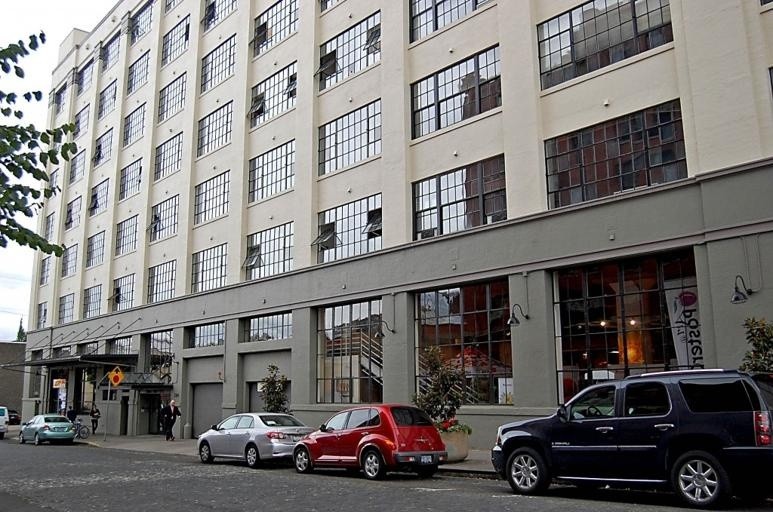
[491,367,773,510]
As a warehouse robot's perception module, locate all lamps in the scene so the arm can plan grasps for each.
[730,274,752,304]
[507,304,528,326]
[375,320,395,339]
[162,359,180,368]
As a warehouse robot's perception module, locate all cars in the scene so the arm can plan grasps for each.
[195,412,316,469]
[291,404,449,479]
[5,409,20,424]
[18,413,75,445]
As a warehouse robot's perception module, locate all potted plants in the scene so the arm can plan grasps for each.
[409,344,472,463]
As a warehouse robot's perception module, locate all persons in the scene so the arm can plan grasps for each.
[66,406,76,424]
[90,405,100,434]
[160,399,181,441]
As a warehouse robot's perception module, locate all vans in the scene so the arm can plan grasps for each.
[0,405,8,439]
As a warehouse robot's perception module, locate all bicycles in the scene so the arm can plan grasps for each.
[72,417,90,439]
